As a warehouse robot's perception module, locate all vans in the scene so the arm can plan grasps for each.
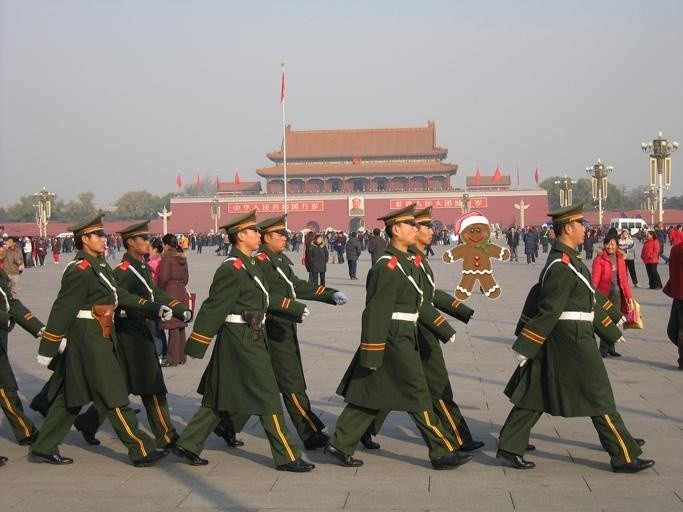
[55,232,74,241]
[606,218,649,238]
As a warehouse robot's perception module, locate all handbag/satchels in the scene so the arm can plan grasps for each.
[624,298,644,330]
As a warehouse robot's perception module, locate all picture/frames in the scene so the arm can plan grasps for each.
[348,196,365,217]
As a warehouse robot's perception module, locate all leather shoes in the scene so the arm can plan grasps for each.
[525,444,535,451]
[277,457,315,472]
[30,400,50,418]
[19,430,39,445]
[613,458,655,473]
[635,438,645,446]
[0,456,8,463]
[133,450,169,467]
[323,440,363,467]
[29,450,73,464]
[431,453,473,470]
[163,433,180,450]
[360,432,380,449]
[303,431,330,450]
[213,423,244,447]
[171,443,208,466]
[73,421,100,446]
[496,448,535,469]
[454,440,484,452]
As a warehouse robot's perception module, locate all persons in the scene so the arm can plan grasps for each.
[588,231,632,362]
[1,221,682,290]
[0,269,47,464]
[323,202,485,471]
[663,242,682,371]
[350,198,363,215]
[145,234,189,368]
[27,214,186,467]
[173,208,349,472]
[496,201,656,472]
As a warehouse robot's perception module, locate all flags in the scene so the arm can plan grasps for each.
[280,66,286,103]
[474,163,539,186]
[176,169,241,190]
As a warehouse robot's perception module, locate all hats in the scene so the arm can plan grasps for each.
[114,219,154,240]
[218,209,261,235]
[547,201,588,224]
[257,213,288,234]
[67,209,106,236]
[414,206,435,227]
[377,202,418,226]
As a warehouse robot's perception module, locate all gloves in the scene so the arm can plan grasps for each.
[332,292,348,305]
[450,334,455,343]
[301,307,310,321]
[515,352,528,368]
[37,291,191,366]
[616,316,627,343]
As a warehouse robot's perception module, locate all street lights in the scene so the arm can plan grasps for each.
[642,188,658,225]
[209,196,220,235]
[553,174,577,209]
[33,185,54,238]
[31,201,43,237]
[585,158,614,224]
[640,130,679,226]
